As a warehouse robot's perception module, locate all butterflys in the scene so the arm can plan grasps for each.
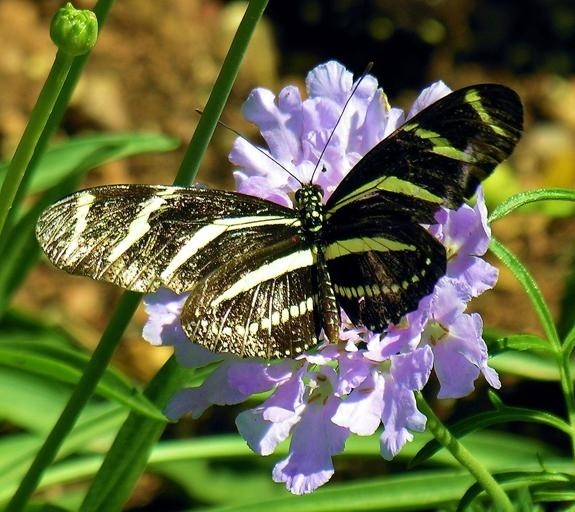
[33,60,524,361]
